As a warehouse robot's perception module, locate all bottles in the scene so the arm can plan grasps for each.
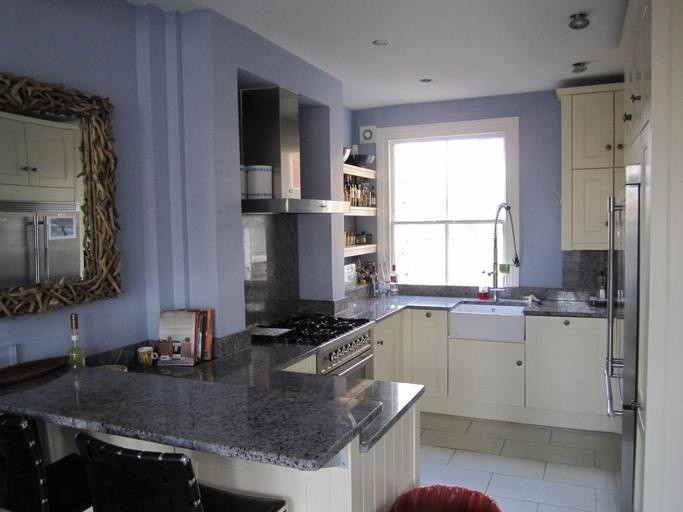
[68,312,86,368]
[344,231,372,246]
[389,264,398,296]
[343,174,377,207]
[599,285,606,300]
[369,265,378,298]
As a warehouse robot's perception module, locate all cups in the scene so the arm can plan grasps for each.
[137,347,158,369]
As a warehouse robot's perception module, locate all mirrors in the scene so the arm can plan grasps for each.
[0,74,124,316]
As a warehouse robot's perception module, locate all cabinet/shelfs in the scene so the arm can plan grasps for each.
[555,83,628,252]
[342,159,380,257]
[375,310,625,441]
[638,120,682,511]
[0,111,82,205]
[617,0,682,149]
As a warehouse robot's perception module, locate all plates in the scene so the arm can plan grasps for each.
[0,355,71,386]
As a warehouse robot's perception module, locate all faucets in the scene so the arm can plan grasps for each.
[487,202,511,288]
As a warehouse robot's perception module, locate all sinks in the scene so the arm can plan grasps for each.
[449,304,525,342]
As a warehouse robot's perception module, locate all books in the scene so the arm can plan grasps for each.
[196,310,206,364]
[157,309,198,366]
[200,308,215,361]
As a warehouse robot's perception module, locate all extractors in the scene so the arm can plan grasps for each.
[241,86,351,215]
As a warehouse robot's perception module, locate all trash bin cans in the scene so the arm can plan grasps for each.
[391,483,503,512]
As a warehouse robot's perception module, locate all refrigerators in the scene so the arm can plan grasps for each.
[604,161,640,512]
[0,203,82,287]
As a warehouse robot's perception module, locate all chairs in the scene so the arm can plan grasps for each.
[0,414,90,512]
[75,432,287,512]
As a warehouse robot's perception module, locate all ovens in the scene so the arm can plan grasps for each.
[316,322,375,380]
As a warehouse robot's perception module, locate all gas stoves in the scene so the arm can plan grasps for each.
[249,312,372,347]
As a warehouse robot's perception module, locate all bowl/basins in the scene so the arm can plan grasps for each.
[343,146,352,163]
[92,364,129,372]
[346,154,376,168]
